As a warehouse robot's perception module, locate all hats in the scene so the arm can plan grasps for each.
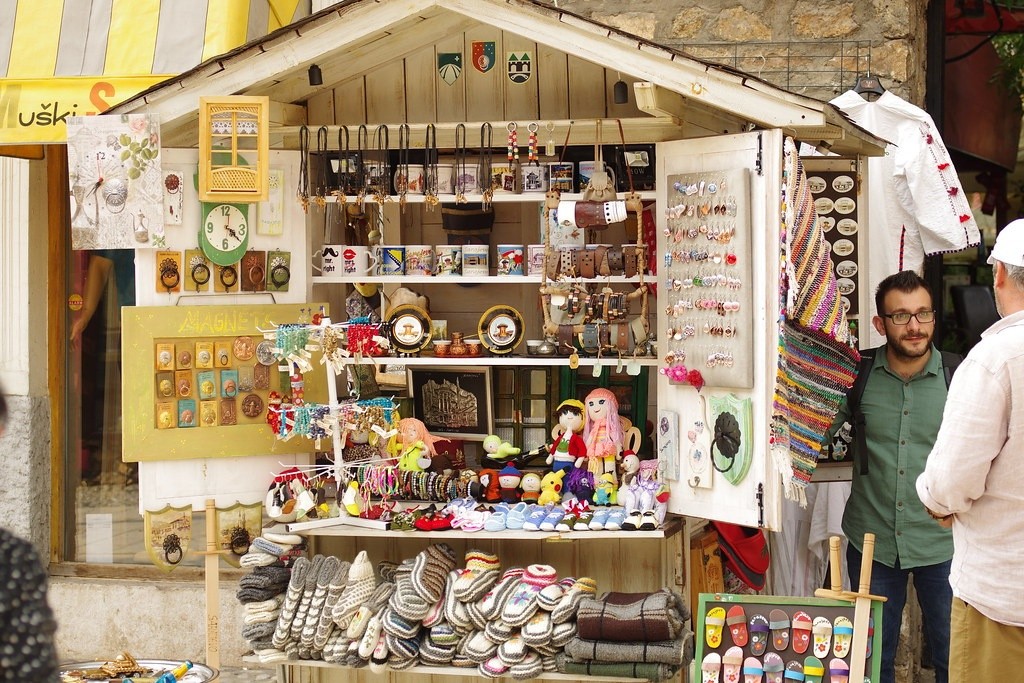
[986,218,1024,268]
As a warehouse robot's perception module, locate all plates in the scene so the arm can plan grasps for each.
[384,304,433,353]
[478,305,525,354]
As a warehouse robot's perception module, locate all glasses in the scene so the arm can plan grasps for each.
[882,311,935,326]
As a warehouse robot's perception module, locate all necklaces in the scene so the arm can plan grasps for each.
[291,118,495,211]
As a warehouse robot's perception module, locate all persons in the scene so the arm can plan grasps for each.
[583,388,623,504]
[545,399,587,496]
[0,384,65,683]
[834,269,963,683]
[914,218,1023,683]
[387,418,450,473]
[71,249,136,346]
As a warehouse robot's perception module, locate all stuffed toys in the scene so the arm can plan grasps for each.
[450,435,642,507]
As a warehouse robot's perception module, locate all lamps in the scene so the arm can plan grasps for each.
[815,139,834,156]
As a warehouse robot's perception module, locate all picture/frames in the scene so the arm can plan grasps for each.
[405,364,494,441]
[121,302,332,463]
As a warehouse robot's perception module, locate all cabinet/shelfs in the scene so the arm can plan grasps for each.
[308,152,658,367]
[257,314,406,532]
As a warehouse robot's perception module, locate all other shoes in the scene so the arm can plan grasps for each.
[391,497,662,532]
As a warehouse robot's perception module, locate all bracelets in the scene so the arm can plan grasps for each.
[276,397,397,438]
[923,505,952,523]
[355,465,467,505]
[273,316,393,357]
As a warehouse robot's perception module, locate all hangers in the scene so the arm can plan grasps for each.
[852,53,886,96]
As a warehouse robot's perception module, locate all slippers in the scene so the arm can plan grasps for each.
[701,605,854,683]
[705,520,770,591]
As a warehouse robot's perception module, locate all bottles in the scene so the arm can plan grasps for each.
[156,660,193,683]
[450,331,468,356]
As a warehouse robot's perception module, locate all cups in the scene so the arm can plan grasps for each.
[393,161,616,195]
[433,340,453,355]
[463,340,482,355]
[311,244,649,276]
[526,340,544,355]
